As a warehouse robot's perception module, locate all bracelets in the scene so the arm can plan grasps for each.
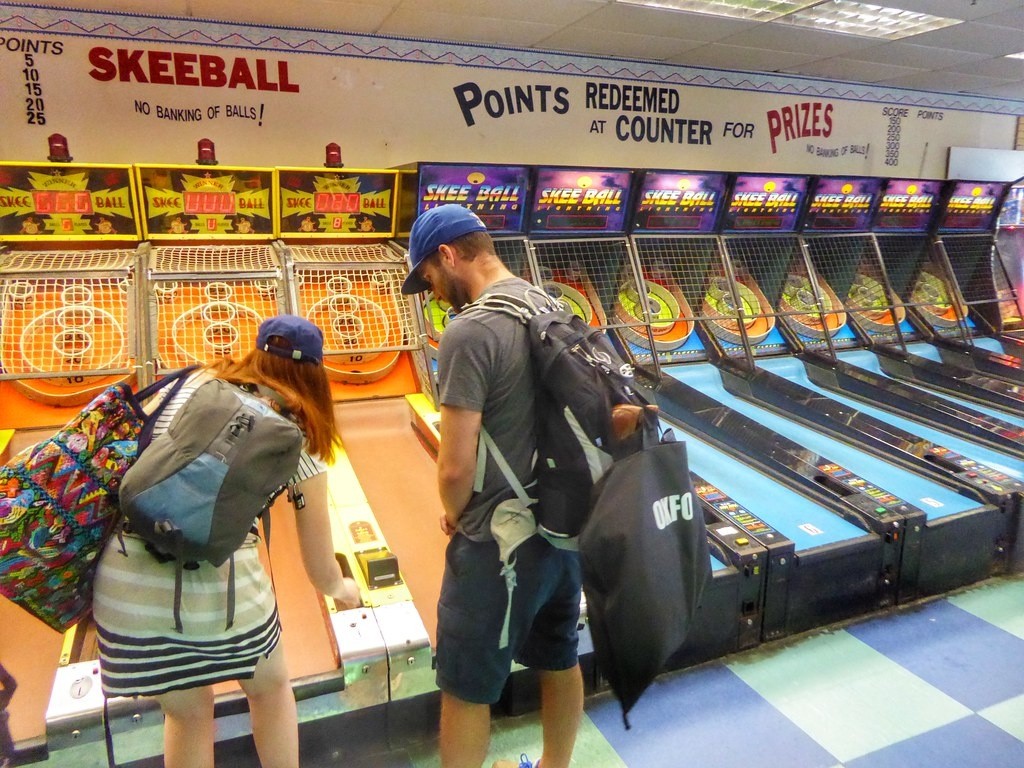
[447,523,455,530]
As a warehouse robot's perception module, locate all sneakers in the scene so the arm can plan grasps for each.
[492,753,540,768]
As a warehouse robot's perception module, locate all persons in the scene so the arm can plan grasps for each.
[92,313,360,768]
[400,203,581,768]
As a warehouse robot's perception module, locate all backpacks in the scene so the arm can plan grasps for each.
[453,287,659,539]
[118,377,302,568]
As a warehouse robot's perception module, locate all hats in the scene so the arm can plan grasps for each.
[256,314,323,365]
[401,203,487,294]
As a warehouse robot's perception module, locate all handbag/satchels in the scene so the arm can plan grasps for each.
[578,410,713,730]
[0,364,201,635]
